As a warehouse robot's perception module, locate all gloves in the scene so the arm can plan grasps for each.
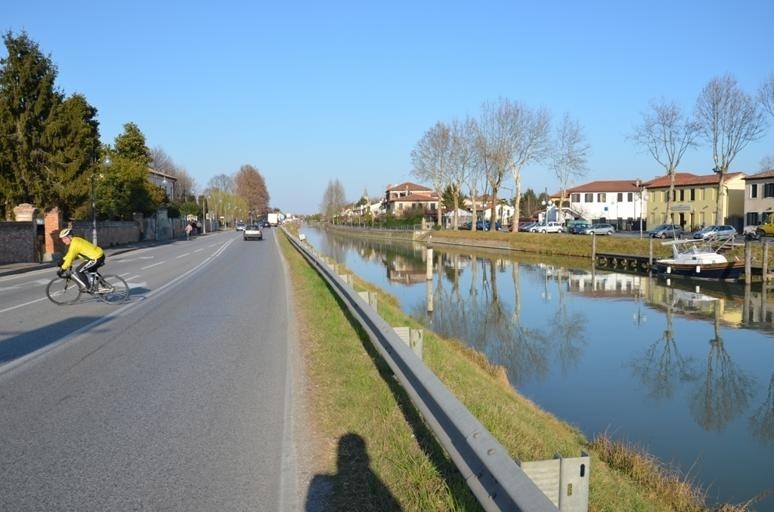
[58,258,66,277]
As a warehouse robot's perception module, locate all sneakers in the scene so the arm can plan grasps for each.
[80,286,95,294]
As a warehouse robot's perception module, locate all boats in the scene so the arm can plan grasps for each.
[656,279,746,308]
[655,219,746,279]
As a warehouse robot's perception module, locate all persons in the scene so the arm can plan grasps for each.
[56,226,112,294]
[184,220,203,241]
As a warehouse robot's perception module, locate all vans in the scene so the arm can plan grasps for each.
[756,213,773,238]
[693,224,738,241]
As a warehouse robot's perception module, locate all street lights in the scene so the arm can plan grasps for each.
[540,187,553,226]
[87,144,111,248]
[630,294,647,332]
[539,267,552,305]
[633,184,649,239]
[182,189,258,235]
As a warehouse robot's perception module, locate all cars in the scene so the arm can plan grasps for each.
[648,224,682,239]
[461,218,616,236]
[235,221,269,241]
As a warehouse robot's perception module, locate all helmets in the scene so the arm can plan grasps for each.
[59,228,72,239]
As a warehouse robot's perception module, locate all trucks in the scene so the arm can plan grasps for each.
[267,213,278,227]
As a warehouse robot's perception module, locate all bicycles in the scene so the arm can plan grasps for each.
[45,259,129,307]
